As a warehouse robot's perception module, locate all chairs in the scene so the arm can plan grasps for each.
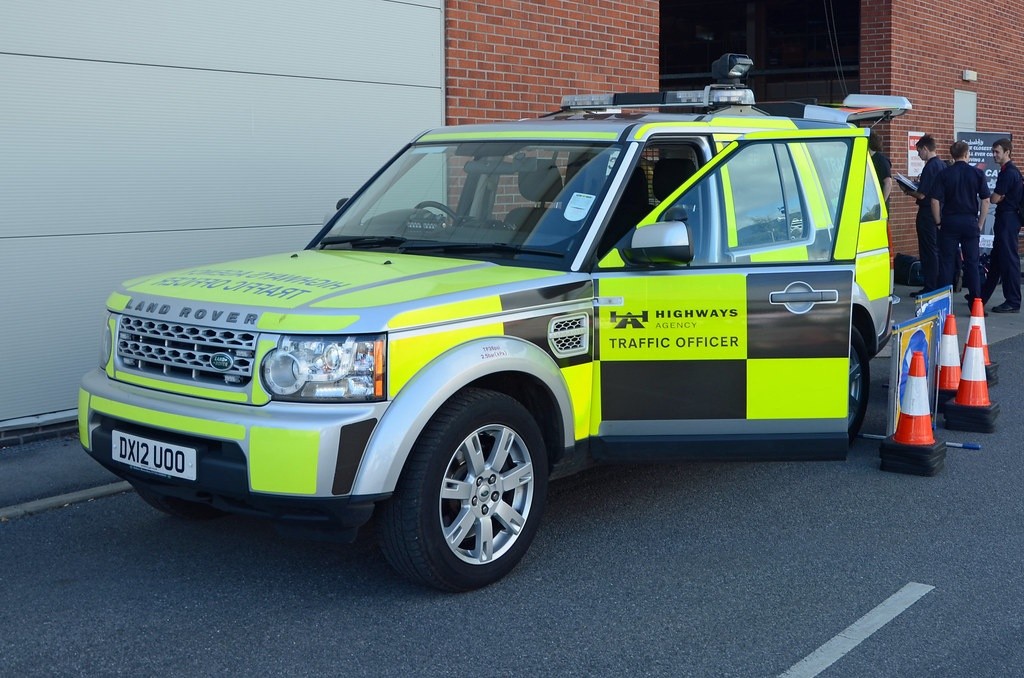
[502,159,564,233]
[598,158,702,264]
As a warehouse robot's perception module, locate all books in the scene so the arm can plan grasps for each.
[894,172,919,191]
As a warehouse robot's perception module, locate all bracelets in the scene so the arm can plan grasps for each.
[904,189,908,195]
[936,223,941,226]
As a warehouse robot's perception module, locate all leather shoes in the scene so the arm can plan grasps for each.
[965,293,969,301]
[991,302,1020,312]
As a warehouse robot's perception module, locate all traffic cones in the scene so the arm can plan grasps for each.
[879,351,947,477]
[944,325,1000,434]
[936,314,961,394]
[961,298,999,386]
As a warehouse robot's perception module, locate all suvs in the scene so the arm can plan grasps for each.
[78,52,912,595]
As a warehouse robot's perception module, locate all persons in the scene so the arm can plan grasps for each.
[868,132,892,218]
[964,139,1024,314]
[930,142,990,316]
[900,134,946,299]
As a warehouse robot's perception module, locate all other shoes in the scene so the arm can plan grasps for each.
[910,288,931,298]
